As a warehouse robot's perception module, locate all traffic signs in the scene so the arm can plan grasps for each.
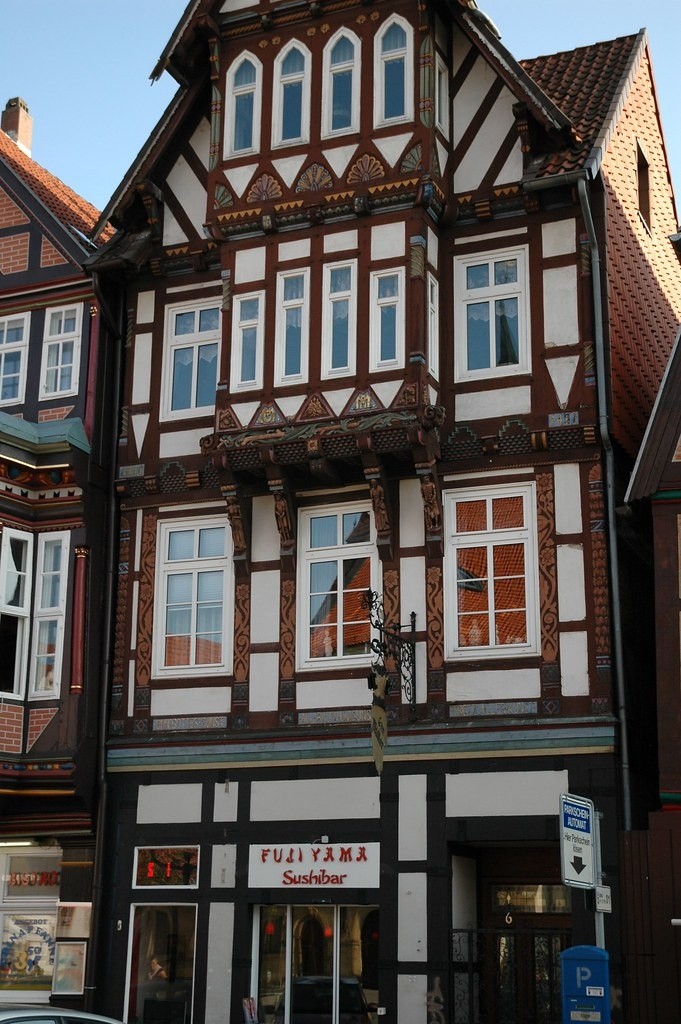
[557,791,598,889]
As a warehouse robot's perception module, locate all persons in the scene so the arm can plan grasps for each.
[144,956,168,1024]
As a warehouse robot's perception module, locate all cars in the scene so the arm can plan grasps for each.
[0,1002,126,1024]
[260,974,379,1024]
